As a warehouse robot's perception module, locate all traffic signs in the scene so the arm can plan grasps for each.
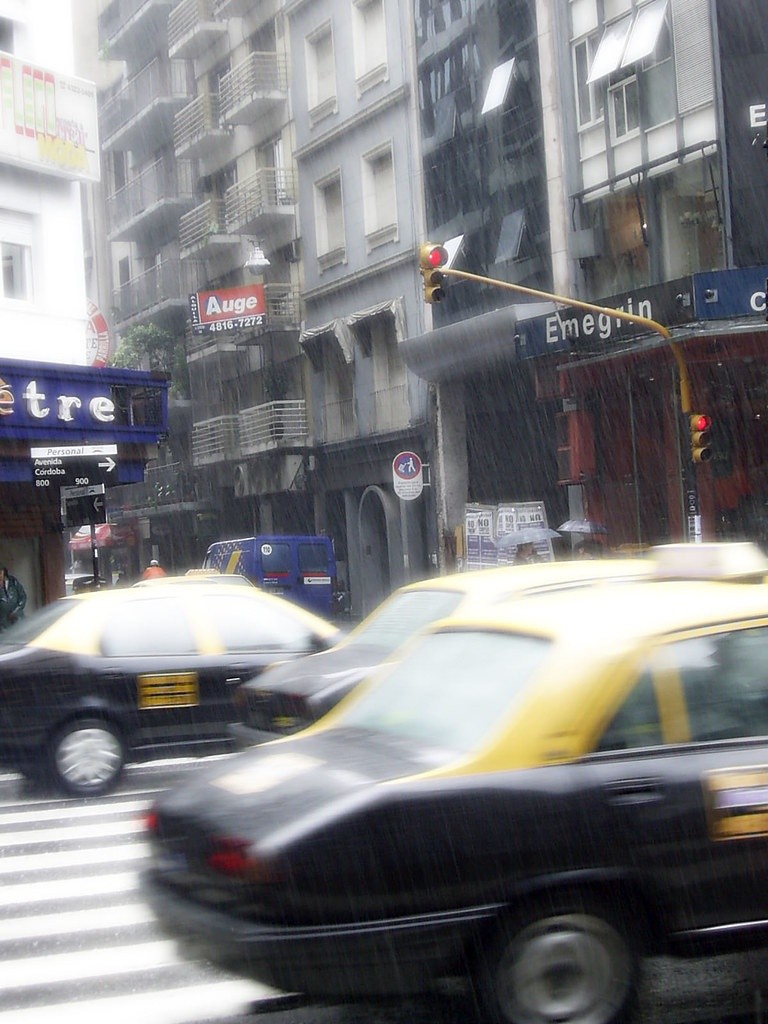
[63,484,107,527]
[31,442,119,488]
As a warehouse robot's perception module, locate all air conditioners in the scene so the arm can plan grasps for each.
[232,455,316,495]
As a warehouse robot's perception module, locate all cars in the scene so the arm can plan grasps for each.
[0,583,346,796]
[229,562,659,750]
[132,575,768,1024]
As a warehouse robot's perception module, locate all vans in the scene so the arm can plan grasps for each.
[202,536,339,627]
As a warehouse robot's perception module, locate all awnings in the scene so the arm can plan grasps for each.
[67,523,137,549]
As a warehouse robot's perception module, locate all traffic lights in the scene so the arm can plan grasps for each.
[420,243,449,304]
[690,414,713,463]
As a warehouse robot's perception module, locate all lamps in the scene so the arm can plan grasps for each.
[244,241,271,276]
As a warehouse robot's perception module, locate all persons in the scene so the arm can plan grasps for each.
[572,532,613,560]
[513,543,544,565]
[0,566,27,628]
[141,560,167,580]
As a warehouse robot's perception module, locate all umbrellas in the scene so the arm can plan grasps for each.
[496,527,562,546]
[556,519,608,532]
